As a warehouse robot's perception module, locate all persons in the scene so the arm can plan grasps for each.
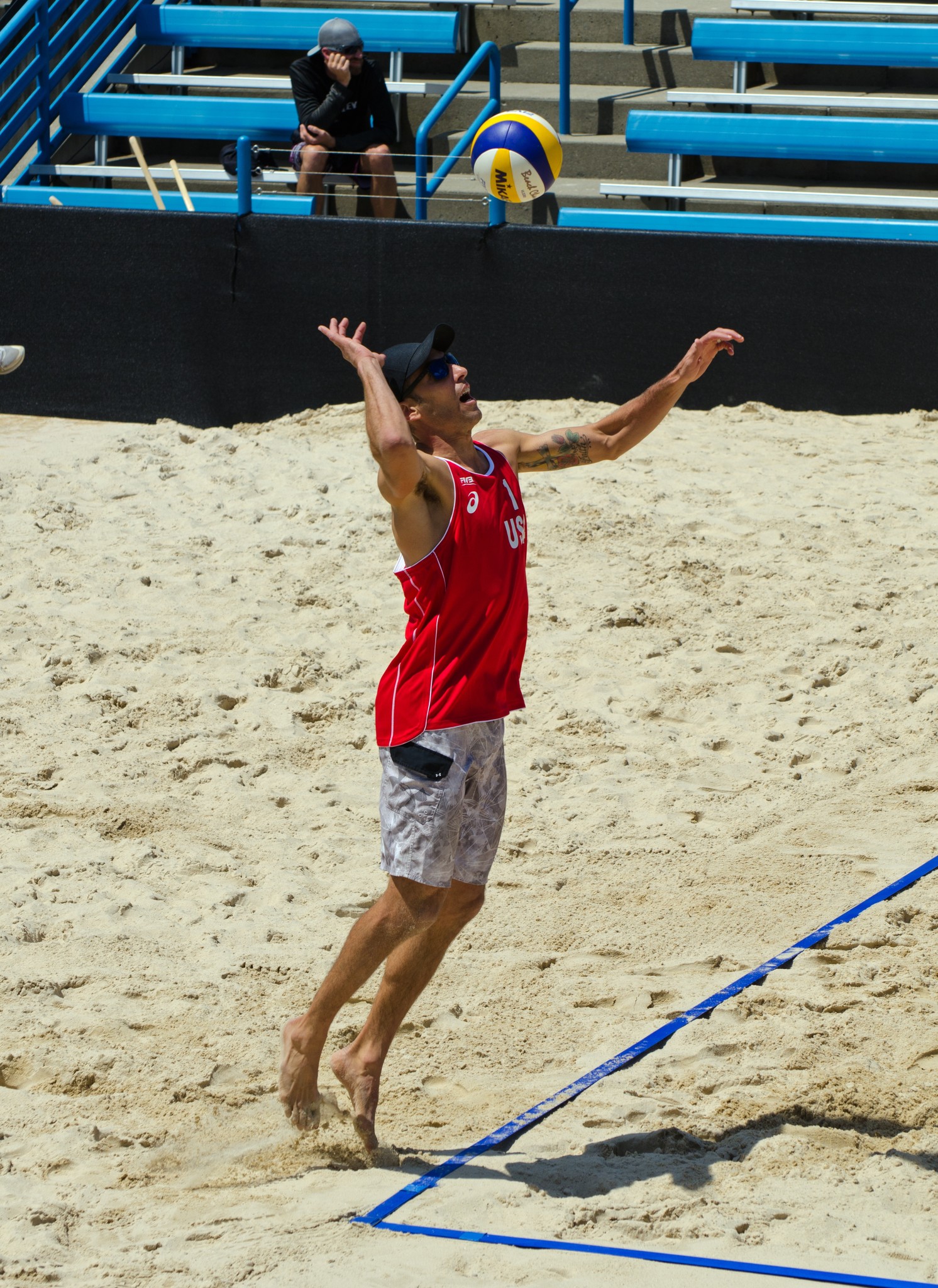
[289,19,399,217]
[278,318,744,1152]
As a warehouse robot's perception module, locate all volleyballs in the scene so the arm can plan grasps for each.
[469,109,563,203]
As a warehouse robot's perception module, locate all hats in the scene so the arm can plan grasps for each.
[306,17,361,57]
[380,323,456,401]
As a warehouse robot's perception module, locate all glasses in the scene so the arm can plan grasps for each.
[324,38,364,55]
[402,352,460,398]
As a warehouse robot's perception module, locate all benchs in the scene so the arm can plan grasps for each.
[550,202,938,246]
[26,90,372,189]
[113,0,467,98]
[715,0,938,25]
[665,15,938,115]
[0,187,314,220]
[599,106,938,214]
